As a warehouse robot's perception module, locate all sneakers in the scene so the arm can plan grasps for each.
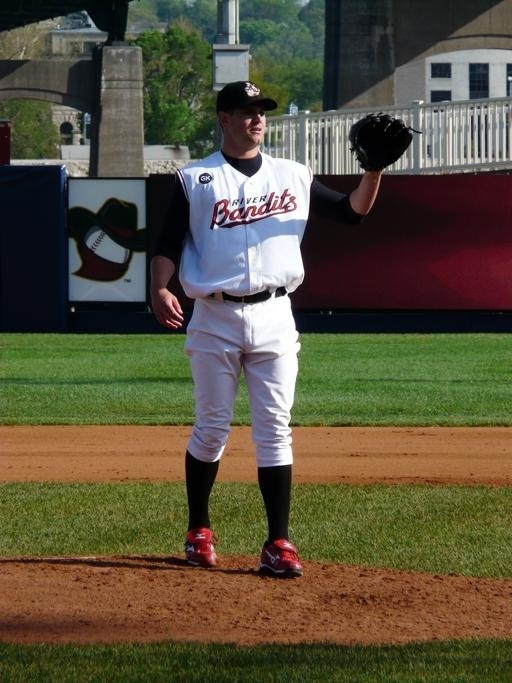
[184,527,220,568]
[259,538,303,577]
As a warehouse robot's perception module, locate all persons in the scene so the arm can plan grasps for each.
[147,79,415,580]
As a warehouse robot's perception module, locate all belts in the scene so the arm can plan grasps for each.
[208,286,287,303]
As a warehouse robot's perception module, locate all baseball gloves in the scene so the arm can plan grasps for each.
[348,110,422,170]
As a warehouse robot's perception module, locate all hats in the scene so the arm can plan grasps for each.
[217,81,277,110]
